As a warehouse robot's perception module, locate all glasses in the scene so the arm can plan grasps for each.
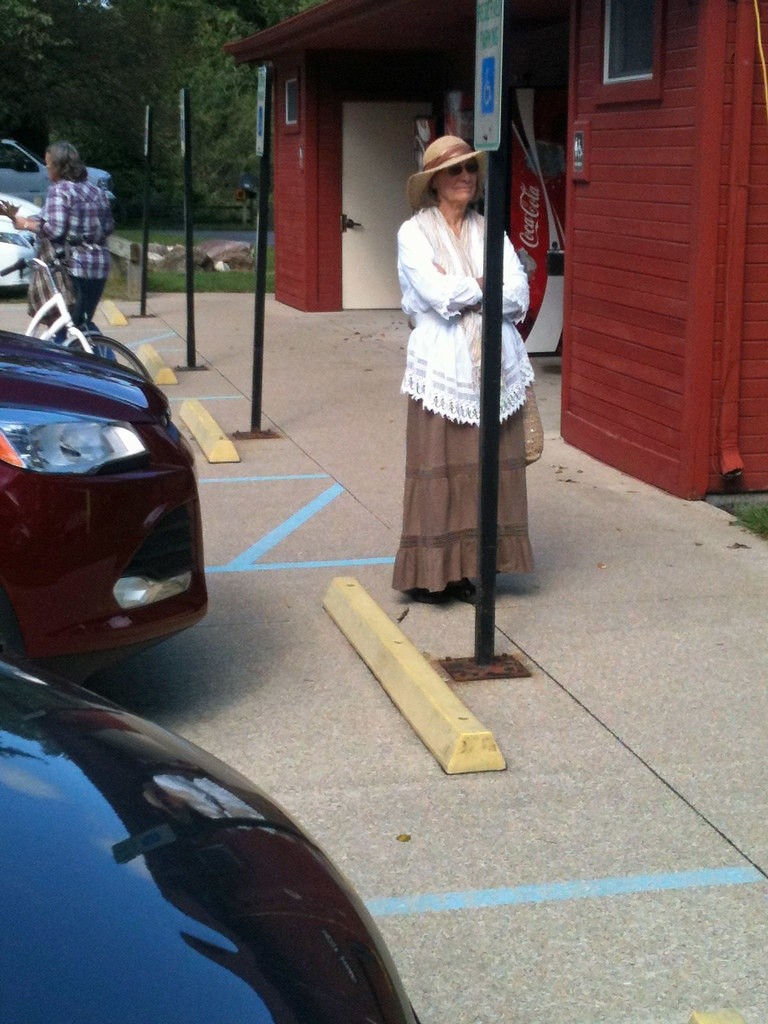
[444,157,479,175]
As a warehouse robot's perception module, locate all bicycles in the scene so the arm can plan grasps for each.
[1,255,154,385]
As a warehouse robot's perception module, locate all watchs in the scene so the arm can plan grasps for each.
[23,221,29,230]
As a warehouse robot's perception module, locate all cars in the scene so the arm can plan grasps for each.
[0,326,206,664]
[0,137,116,208]
[0,193,47,287]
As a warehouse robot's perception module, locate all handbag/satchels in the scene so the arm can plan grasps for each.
[523,380,544,466]
[24,236,78,323]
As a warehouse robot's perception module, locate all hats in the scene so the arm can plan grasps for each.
[408,135,489,210]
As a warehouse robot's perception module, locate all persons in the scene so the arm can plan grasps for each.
[13,139,119,361]
[391,134,534,605]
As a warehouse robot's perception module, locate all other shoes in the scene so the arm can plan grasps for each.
[411,578,479,604]
